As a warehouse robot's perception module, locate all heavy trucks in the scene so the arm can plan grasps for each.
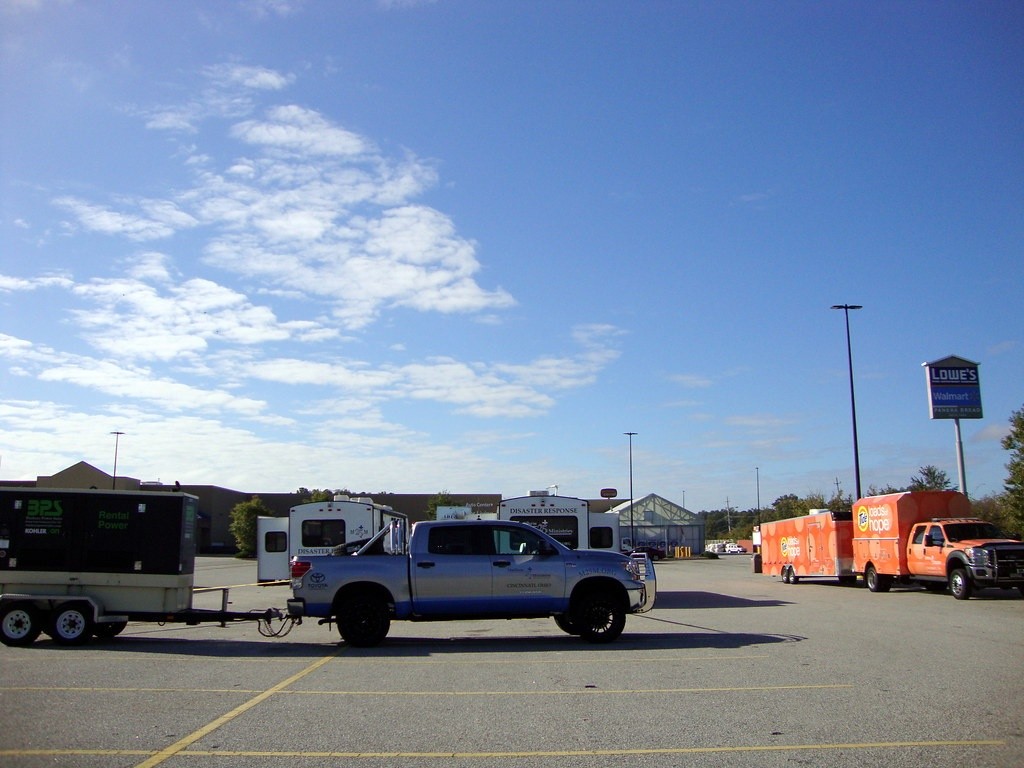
[753,489,1024,600]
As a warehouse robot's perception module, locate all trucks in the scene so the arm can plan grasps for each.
[497,489,633,557]
[256,494,410,588]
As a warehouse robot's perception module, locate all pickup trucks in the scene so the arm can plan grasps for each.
[287,519,647,649]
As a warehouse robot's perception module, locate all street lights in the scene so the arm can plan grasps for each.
[622,431,639,548]
[109,431,126,490]
[755,467,760,531]
[830,303,863,501]
[683,490,685,508]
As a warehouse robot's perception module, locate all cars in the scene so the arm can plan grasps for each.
[704,540,747,554]
[621,546,665,561]
